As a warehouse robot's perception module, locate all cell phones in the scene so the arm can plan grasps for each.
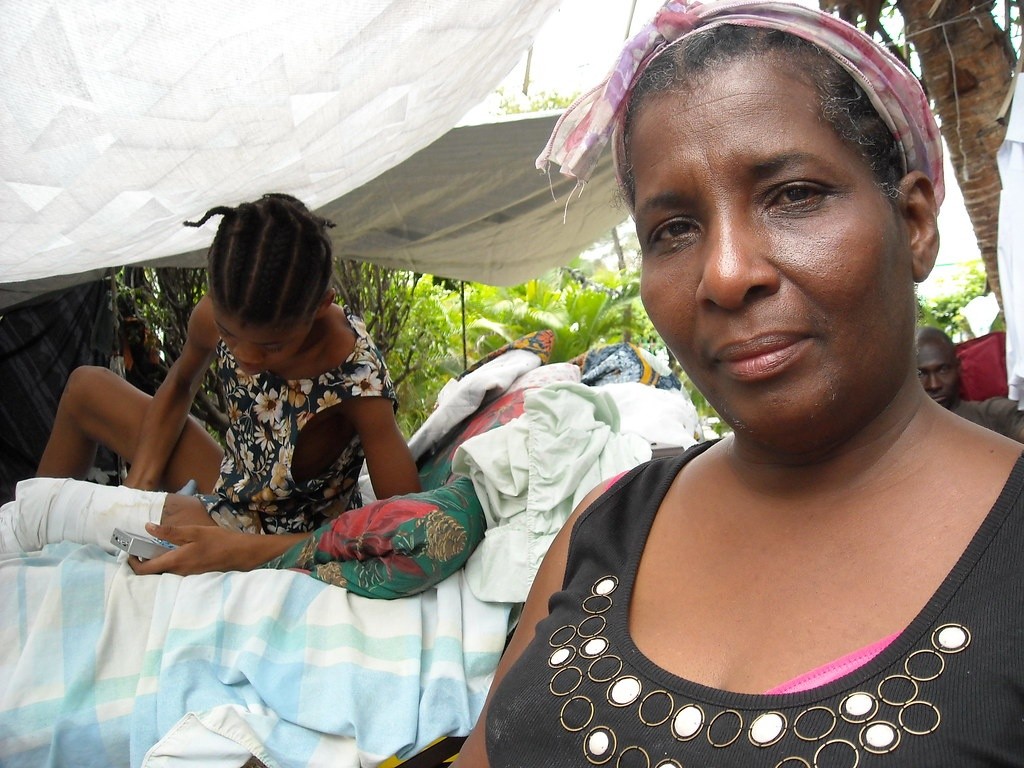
[110,528,177,559]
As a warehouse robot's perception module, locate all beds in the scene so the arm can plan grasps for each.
[1,345,706,768]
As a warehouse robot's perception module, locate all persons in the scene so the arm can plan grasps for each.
[448,0,1024,768]
[915,327,1024,443]
[36,193,422,575]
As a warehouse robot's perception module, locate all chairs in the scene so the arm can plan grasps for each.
[954,333,1008,402]
